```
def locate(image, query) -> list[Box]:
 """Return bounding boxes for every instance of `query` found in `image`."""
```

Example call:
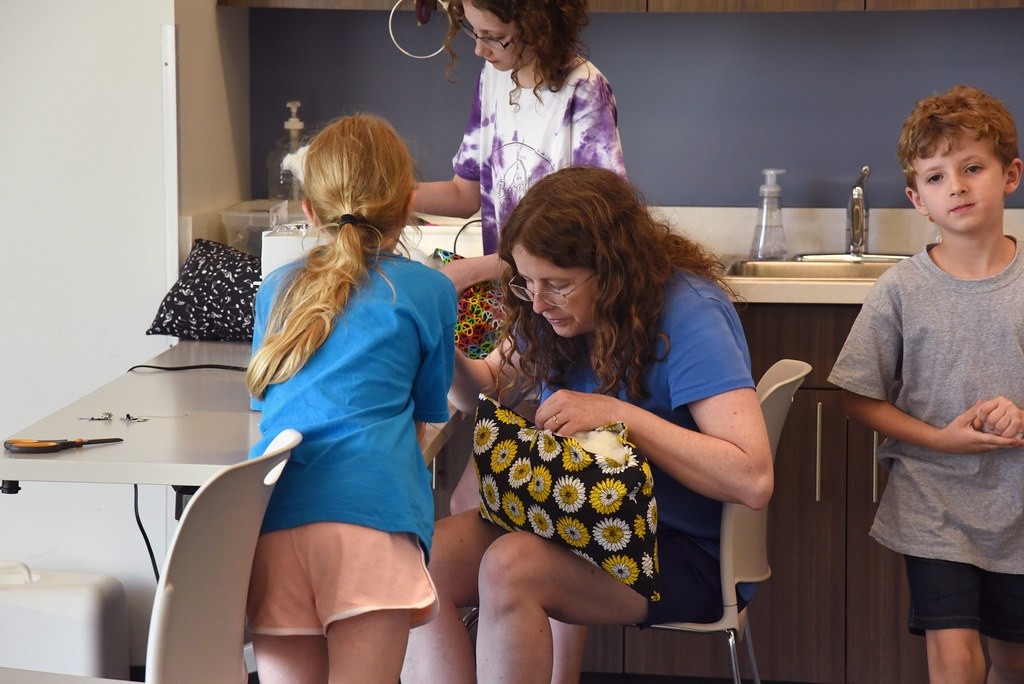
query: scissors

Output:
[4,437,123,454]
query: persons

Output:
[825,83,1024,684]
[246,113,459,683]
[398,167,774,684]
[404,0,630,684]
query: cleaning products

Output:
[265,100,320,233]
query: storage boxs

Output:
[220,198,303,255]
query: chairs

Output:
[0,428,301,684]
[633,358,812,684]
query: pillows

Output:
[144,237,261,341]
[473,393,662,602]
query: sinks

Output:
[722,258,900,281]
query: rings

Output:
[552,415,562,426]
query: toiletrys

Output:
[749,169,788,261]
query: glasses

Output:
[457,12,516,50]
[509,272,599,306]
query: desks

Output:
[0,341,465,494]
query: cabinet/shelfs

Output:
[579,303,929,684]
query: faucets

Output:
[844,165,870,257]
